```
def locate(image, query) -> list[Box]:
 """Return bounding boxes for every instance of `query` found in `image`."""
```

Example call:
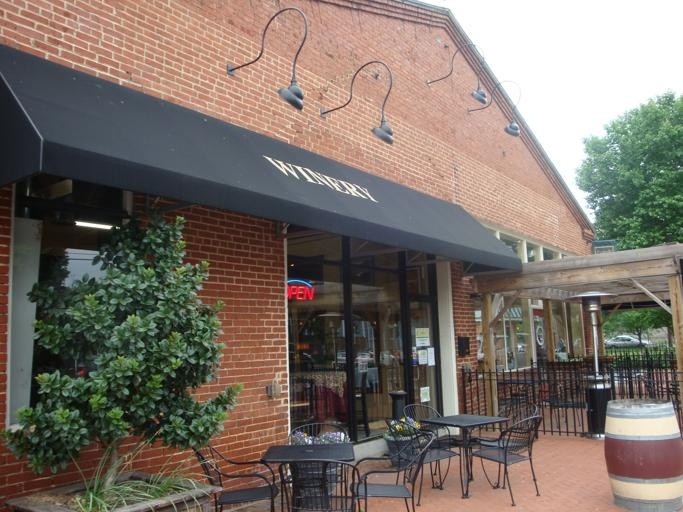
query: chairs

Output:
[350,433,435,511]
[384,403,542,507]
[192,421,368,511]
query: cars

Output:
[604,335,647,349]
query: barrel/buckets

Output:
[604,398,682,512]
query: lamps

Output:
[319,61,394,145]
[426,42,487,106]
[467,80,521,136]
[227,7,307,111]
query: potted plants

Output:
[0,196,222,512]
